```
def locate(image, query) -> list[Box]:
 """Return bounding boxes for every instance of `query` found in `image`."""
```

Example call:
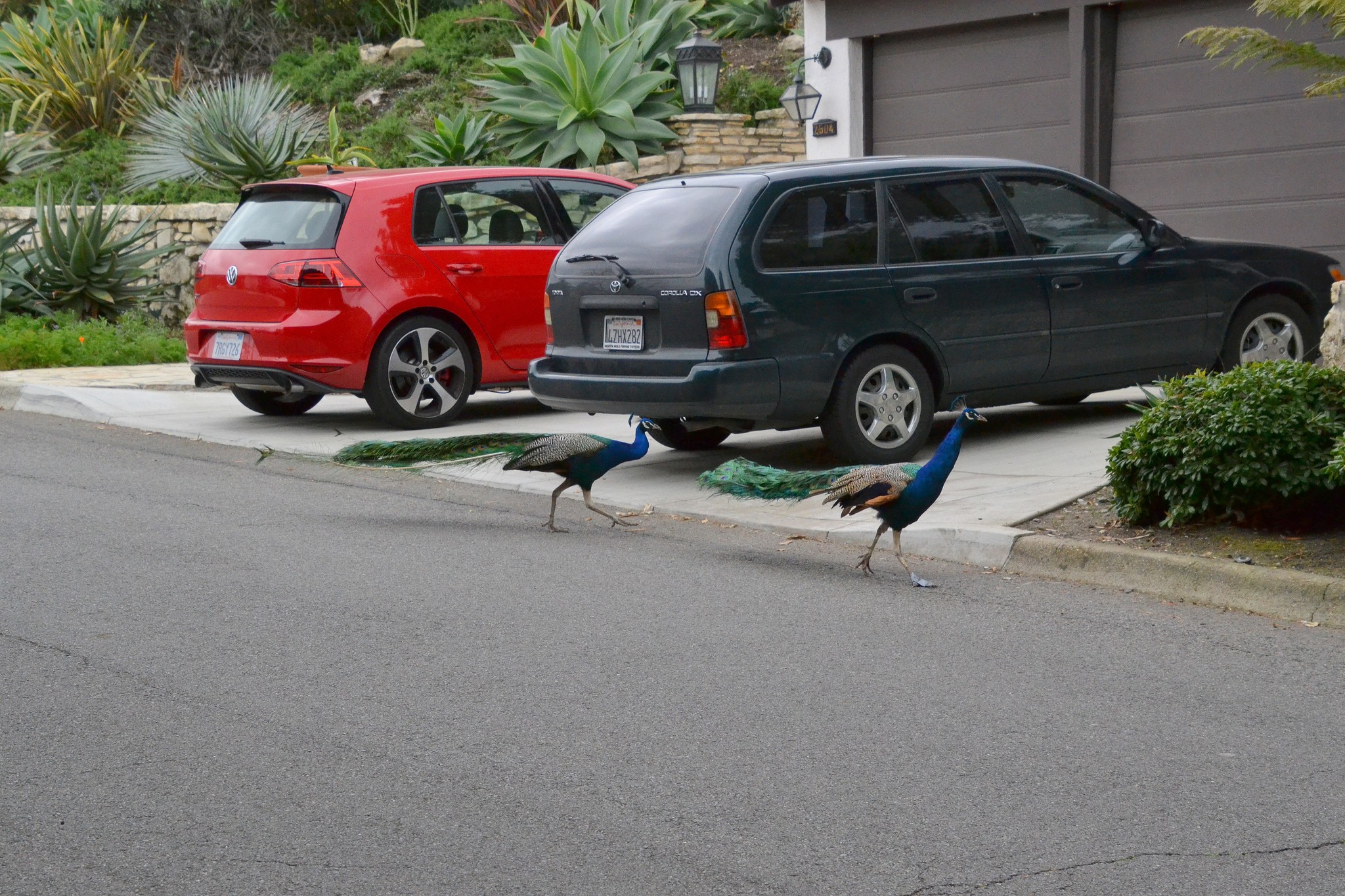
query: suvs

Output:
[526,152,1345,467]
[181,163,639,430]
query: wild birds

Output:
[695,395,988,588]
[254,409,661,533]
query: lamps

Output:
[777,47,833,124]
[670,27,726,115]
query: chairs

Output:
[482,206,526,246]
[301,208,332,238]
[431,201,472,242]
